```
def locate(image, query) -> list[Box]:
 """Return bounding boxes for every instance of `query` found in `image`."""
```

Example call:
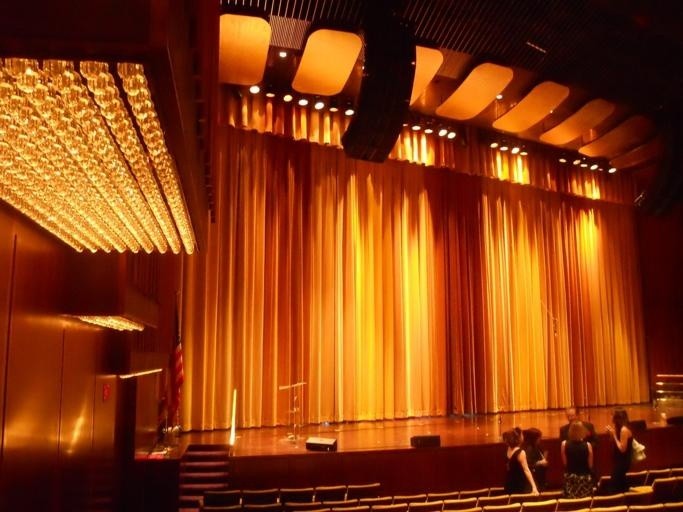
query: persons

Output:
[560,419,595,499]
[561,406,600,461]
[503,427,540,496]
[603,407,633,493]
[523,428,548,493]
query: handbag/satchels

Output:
[632,439,646,463]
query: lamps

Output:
[266,81,528,156]
[558,151,616,173]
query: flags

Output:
[174,308,184,412]
